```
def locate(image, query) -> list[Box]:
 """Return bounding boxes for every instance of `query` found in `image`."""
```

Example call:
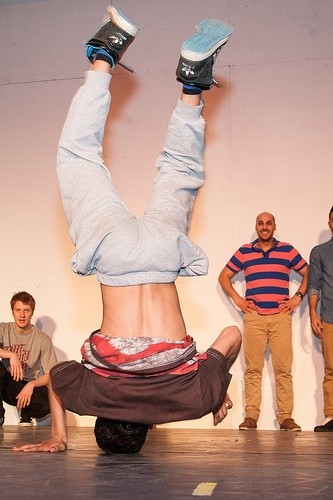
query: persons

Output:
[308,206,333,432]
[11,6,242,455]
[0,292,58,426]
[219,213,308,431]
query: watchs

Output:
[295,292,303,299]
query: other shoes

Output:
[17,408,34,427]
[280,418,303,431]
[174,18,234,90]
[238,418,258,430]
[0,418,5,427]
[314,420,333,432]
[85,4,140,70]
